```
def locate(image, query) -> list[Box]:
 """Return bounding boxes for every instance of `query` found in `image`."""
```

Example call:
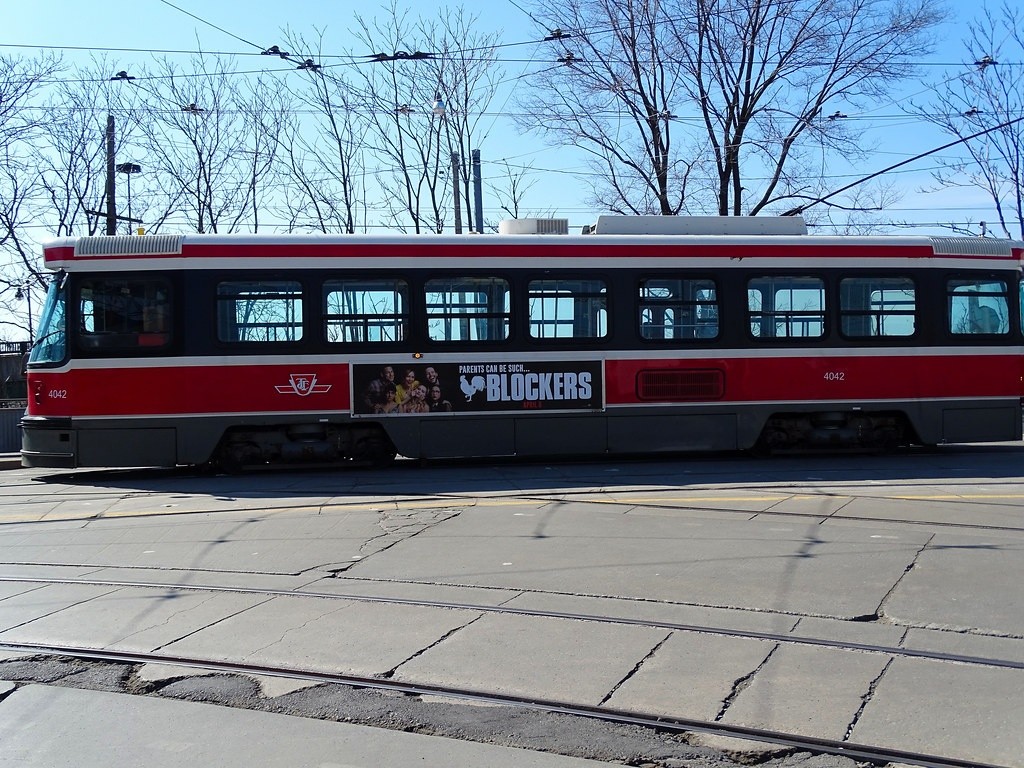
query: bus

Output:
[16,117,1024,472]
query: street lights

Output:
[116,162,142,235]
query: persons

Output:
[358,365,451,415]
